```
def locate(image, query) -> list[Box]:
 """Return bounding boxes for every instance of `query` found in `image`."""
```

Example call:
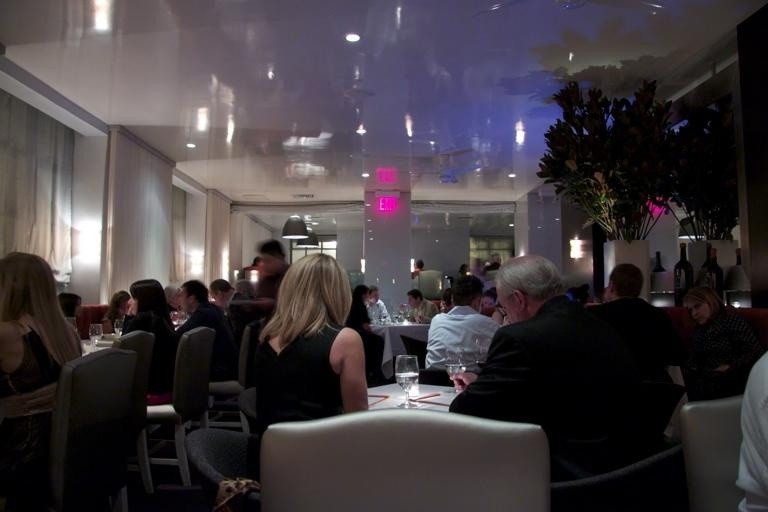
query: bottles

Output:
[652,250,666,272]
[699,241,724,301]
[673,243,693,306]
[736,247,741,265]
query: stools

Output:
[551,444,682,488]
[184,428,260,501]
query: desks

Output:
[80,334,118,356]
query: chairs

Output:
[258,410,552,512]
[679,396,742,512]
[112,330,155,495]
[147,327,216,487]
[209,318,263,432]
[47,347,138,512]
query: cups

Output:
[65,317,78,332]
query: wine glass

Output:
[443,346,468,377]
[114,319,125,336]
[393,353,421,409]
[88,324,104,351]
[373,303,424,325]
[471,335,493,366]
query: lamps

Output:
[281,216,319,246]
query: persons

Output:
[411,260,424,279]
[121,279,177,406]
[58,293,82,323]
[346,284,391,335]
[238,253,368,435]
[425,275,510,374]
[165,287,181,312]
[210,238,290,343]
[736,352,767,512]
[450,254,638,512]
[406,289,439,322]
[0,252,82,498]
[583,264,688,379]
[682,286,767,403]
[101,291,131,334]
[180,281,239,395]
